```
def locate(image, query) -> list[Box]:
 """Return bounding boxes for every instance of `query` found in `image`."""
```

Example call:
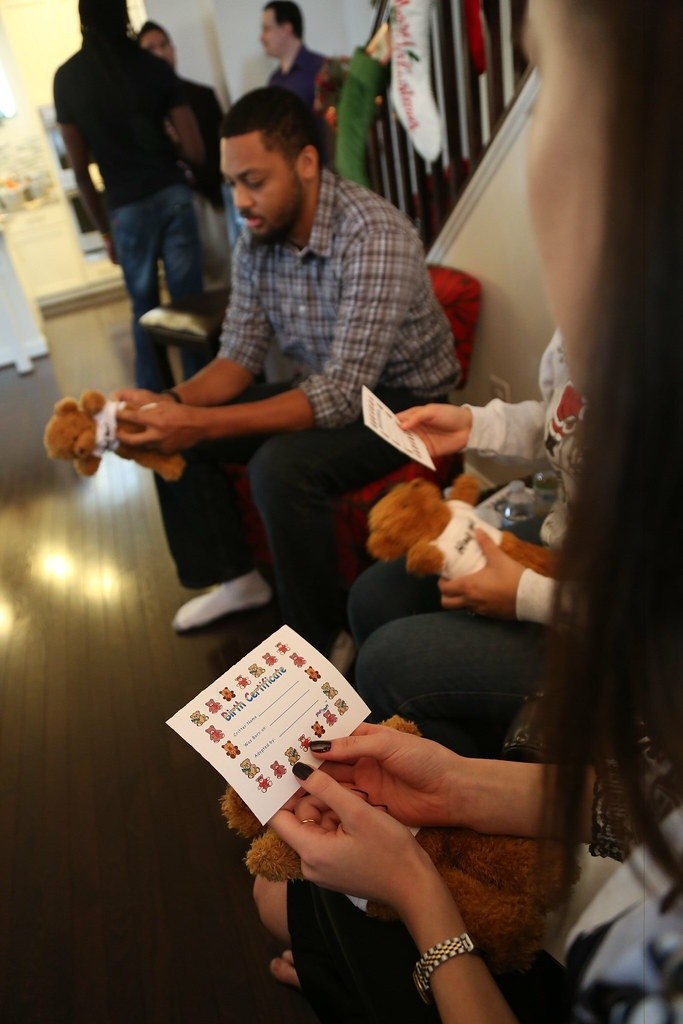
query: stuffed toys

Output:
[217,710,575,952]
[365,477,564,633]
[45,389,193,482]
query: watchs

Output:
[414,931,478,1005]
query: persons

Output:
[54,0,225,394]
[140,21,224,290]
[260,0,345,128]
[250,2,683,1022]
[131,86,467,635]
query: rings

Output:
[299,817,317,825]
